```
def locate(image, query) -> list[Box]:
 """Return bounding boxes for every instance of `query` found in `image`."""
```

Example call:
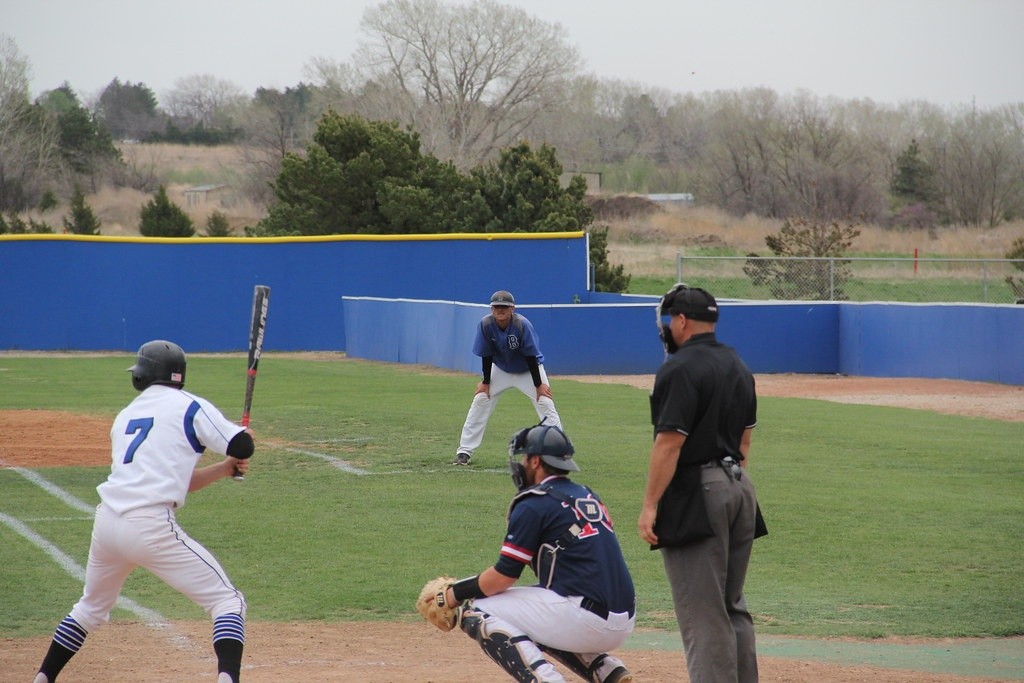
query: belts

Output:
[710,459,737,467]
[581,598,634,621]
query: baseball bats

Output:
[232,284,271,482]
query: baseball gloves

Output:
[415,575,461,632]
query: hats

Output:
[663,289,718,322]
[489,290,515,306]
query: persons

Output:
[453,289,562,467]
[638,282,769,683]
[32,340,255,683]
[415,416,637,683]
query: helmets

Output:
[523,426,581,472]
[126,340,186,390]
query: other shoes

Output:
[603,666,633,683]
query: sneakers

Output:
[453,453,470,465]
